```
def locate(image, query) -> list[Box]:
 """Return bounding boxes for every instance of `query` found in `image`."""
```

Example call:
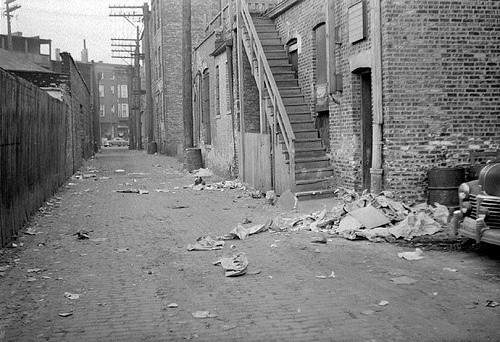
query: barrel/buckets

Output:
[187,148,202,173]
[427,167,465,215]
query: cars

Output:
[107,138,129,147]
[450,162,499,246]
